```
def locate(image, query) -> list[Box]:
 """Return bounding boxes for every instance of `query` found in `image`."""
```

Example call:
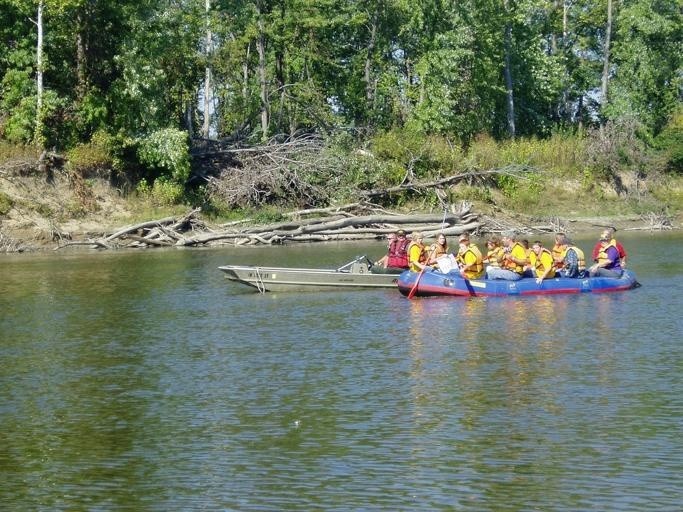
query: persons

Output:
[374,229,627,284]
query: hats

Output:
[397,230,406,236]
[459,234,469,242]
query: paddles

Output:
[408,248,434,298]
[460,272,486,289]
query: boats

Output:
[395,266,641,298]
[217,262,405,294]
